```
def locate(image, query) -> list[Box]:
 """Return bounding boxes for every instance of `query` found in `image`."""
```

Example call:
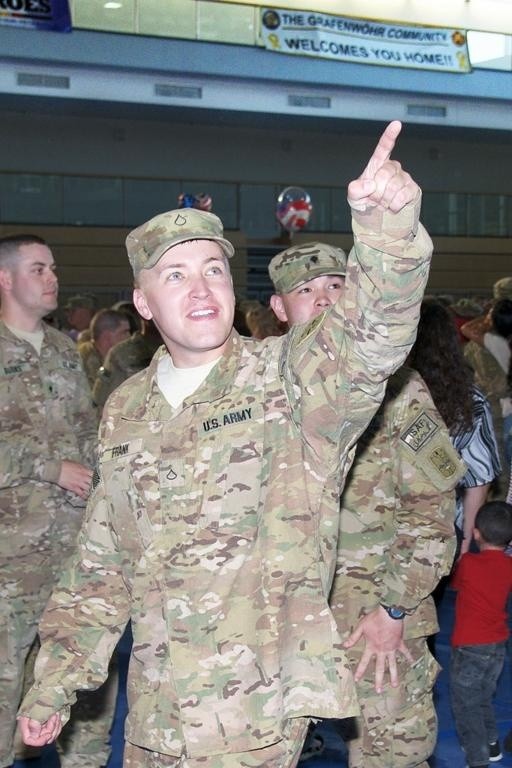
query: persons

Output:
[405,275,511,767]
[15,120,459,768]
[44,295,284,407]
[1,234,119,767]
[268,244,459,767]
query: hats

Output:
[124,207,235,271]
[493,278,512,300]
[64,298,96,310]
[268,242,346,296]
[449,299,483,318]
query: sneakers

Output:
[299,734,324,761]
[487,741,503,763]
